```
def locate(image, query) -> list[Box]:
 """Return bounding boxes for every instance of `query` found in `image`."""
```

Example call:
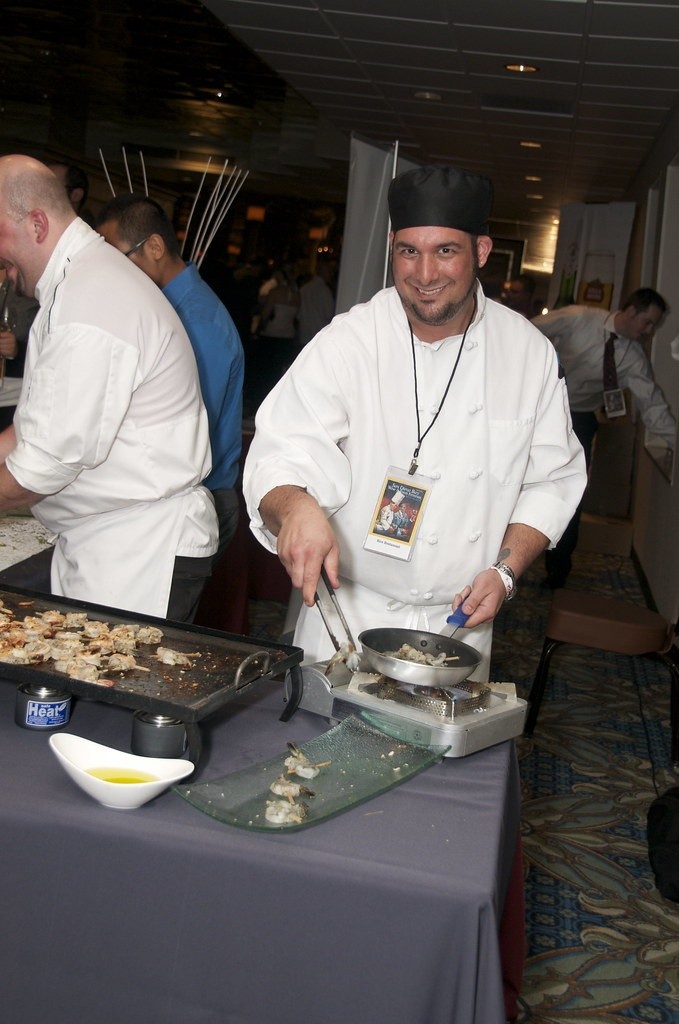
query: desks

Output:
[0,664,521,1023]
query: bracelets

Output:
[493,561,517,601]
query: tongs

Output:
[314,565,358,673]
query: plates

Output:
[170,712,452,834]
[48,733,195,809]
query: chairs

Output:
[520,588,679,763]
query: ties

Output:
[603,332,620,422]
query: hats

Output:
[387,165,492,237]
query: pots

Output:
[357,603,485,688]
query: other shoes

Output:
[541,566,570,591]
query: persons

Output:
[510,275,536,315]
[255,251,338,369]
[241,168,586,687]
[530,288,677,595]
[374,490,417,538]
[94,195,245,621]
[0,162,88,431]
[0,154,219,626]
